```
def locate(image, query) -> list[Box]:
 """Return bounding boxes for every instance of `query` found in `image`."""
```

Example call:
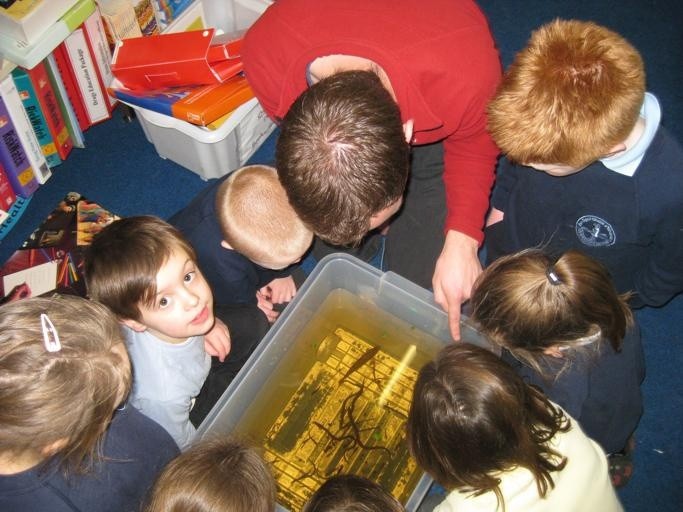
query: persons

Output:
[166,162,316,325]
[407,338,624,511]
[304,472,409,512]
[239,0,505,342]
[138,431,282,511]
[0,291,183,512]
[80,211,232,450]
[479,17,683,311]
[468,244,647,456]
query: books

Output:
[1,0,255,212]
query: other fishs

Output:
[312,359,393,477]
[338,345,381,386]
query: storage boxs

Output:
[183,251,500,509]
[125,1,285,186]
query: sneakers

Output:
[607,436,634,489]
